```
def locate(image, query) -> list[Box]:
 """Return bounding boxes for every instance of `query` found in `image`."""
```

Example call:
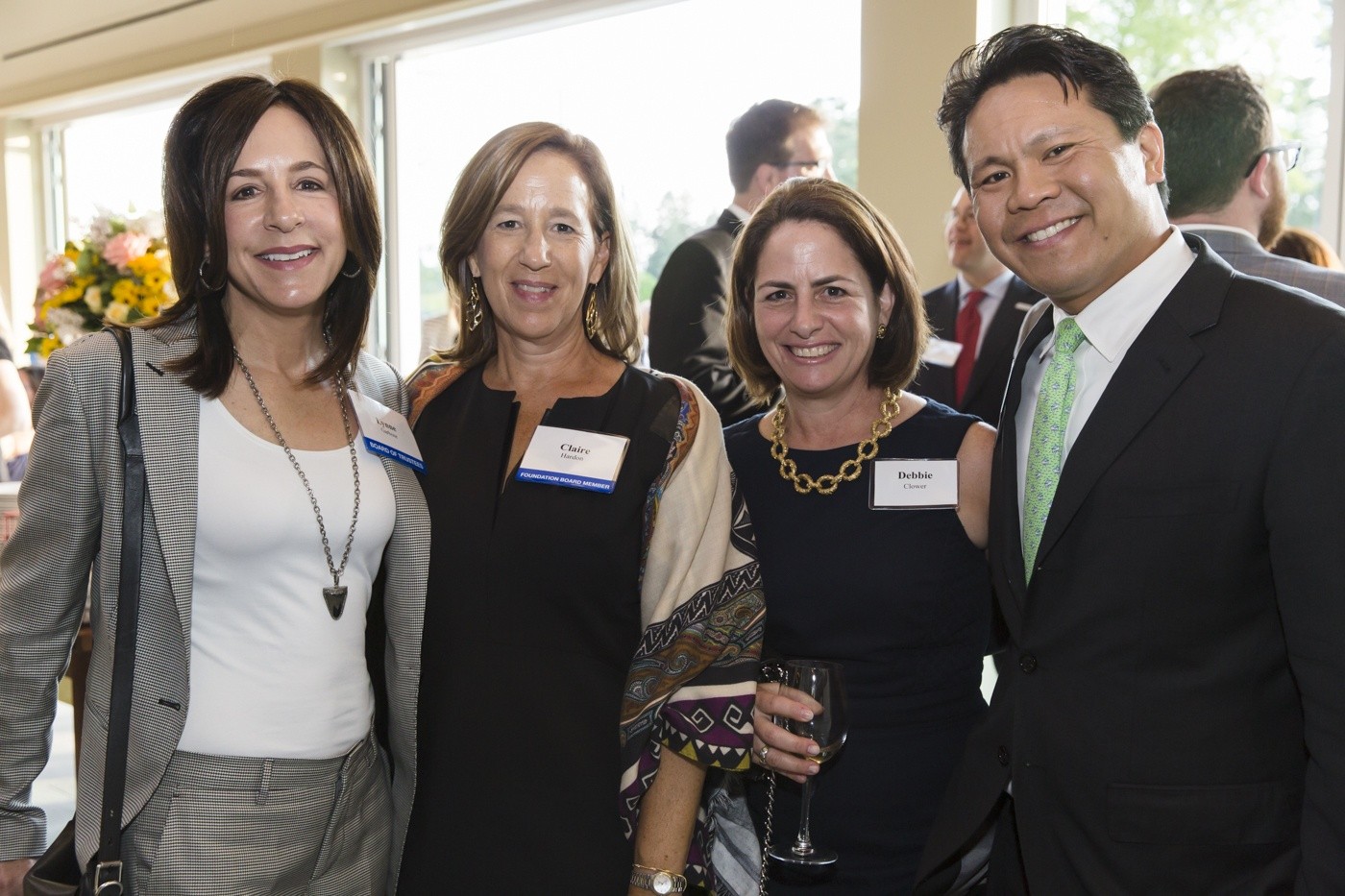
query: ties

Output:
[953,291,988,409]
[1021,316,1087,587]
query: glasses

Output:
[770,159,833,176]
[1244,143,1300,179]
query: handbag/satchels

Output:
[21,813,127,896]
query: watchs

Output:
[630,862,688,896]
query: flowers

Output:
[27,211,180,368]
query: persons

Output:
[0,77,433,896]
[649,100,840,416]
[900,183,1043,424]
[695,177,1019,895]
[404,119,767,896]
[933,21,1345,896]
[1145,64,1345,307]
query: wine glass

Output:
[764,660,848,866]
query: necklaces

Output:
[767,380,903,501]
[230,316,360,624]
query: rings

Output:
[758,744,770,765]
